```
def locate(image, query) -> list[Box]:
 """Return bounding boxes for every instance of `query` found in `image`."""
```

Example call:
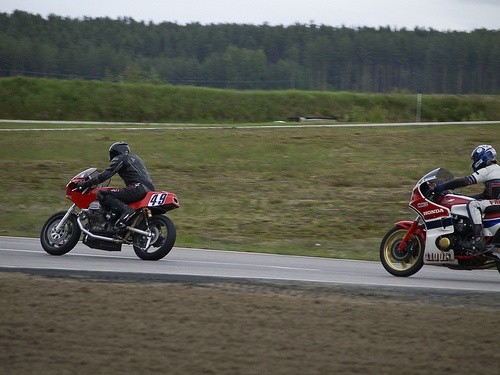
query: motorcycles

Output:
[379,167,500,277]
[40,168,180,260]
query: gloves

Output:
[426,183,443,195]
[77,180,93,192]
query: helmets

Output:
[470,144,497,172]
[108,142,131,160]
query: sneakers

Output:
[461,232,484,247]
[115,207,135,227]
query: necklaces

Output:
[426,143,499,250]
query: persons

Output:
[71,140,154,229]
